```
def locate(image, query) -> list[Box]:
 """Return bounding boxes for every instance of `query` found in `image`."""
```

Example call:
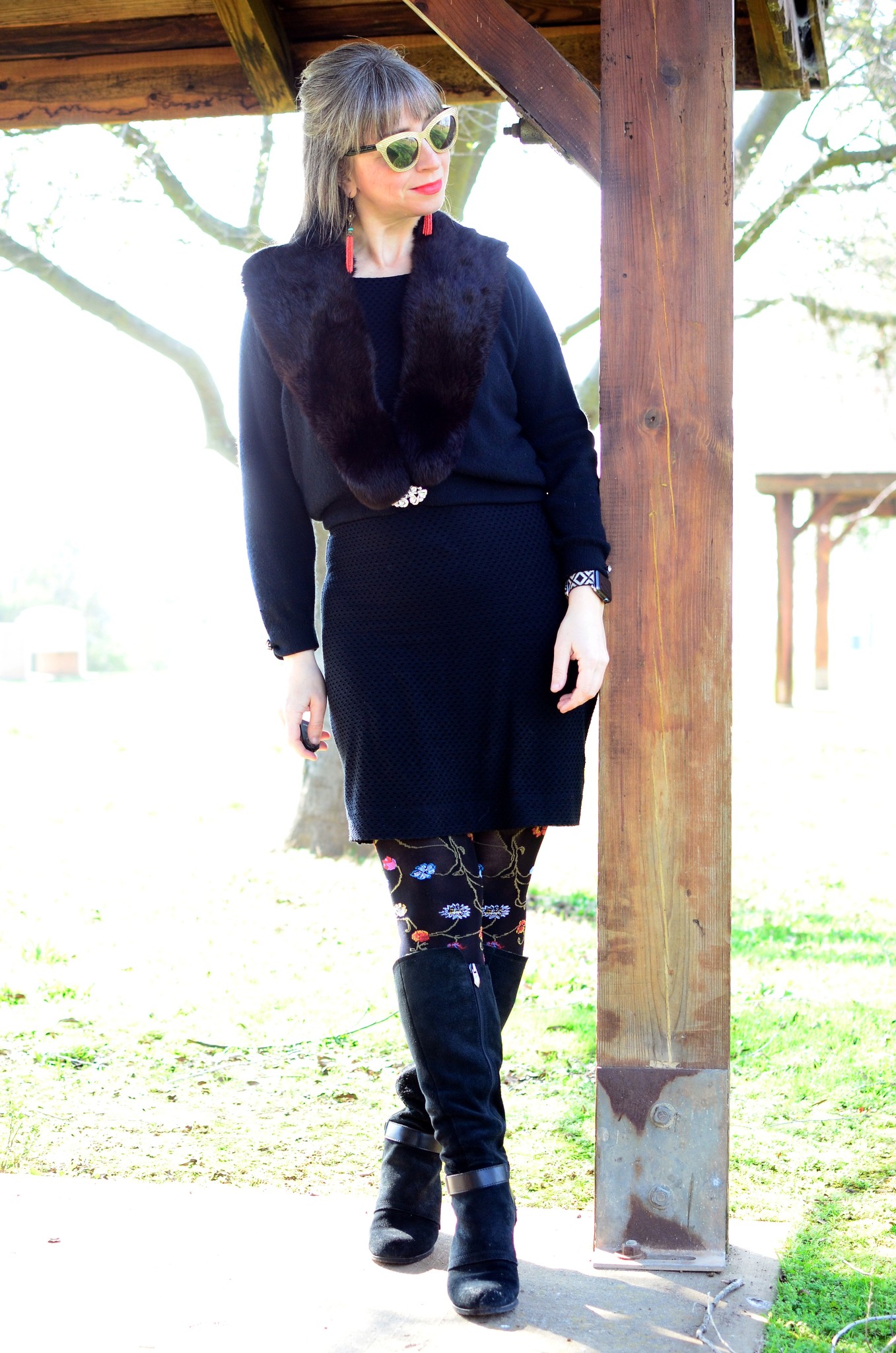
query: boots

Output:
[372,944,526,1265]
[393,944,521,1316]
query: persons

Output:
[238,43,612,1321]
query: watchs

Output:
[565,570,612,604]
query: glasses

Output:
[341,104,460,173]
